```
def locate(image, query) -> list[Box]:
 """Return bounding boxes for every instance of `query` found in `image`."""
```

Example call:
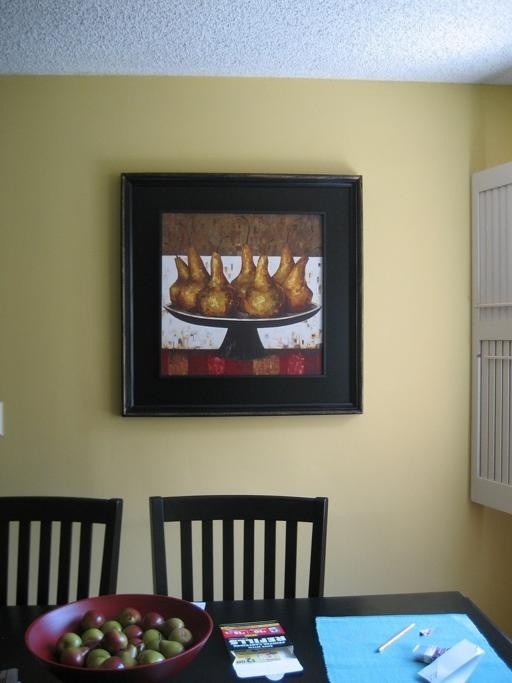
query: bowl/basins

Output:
[23,594,215,681]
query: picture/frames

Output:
[120,171,362,417]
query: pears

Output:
[170,243,313,317]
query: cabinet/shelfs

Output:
[470,160,512,515]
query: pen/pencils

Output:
[377,623,415,653]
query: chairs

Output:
[148,495,329,601]
[0,496,123,606]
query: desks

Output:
[0,591,512,683]
